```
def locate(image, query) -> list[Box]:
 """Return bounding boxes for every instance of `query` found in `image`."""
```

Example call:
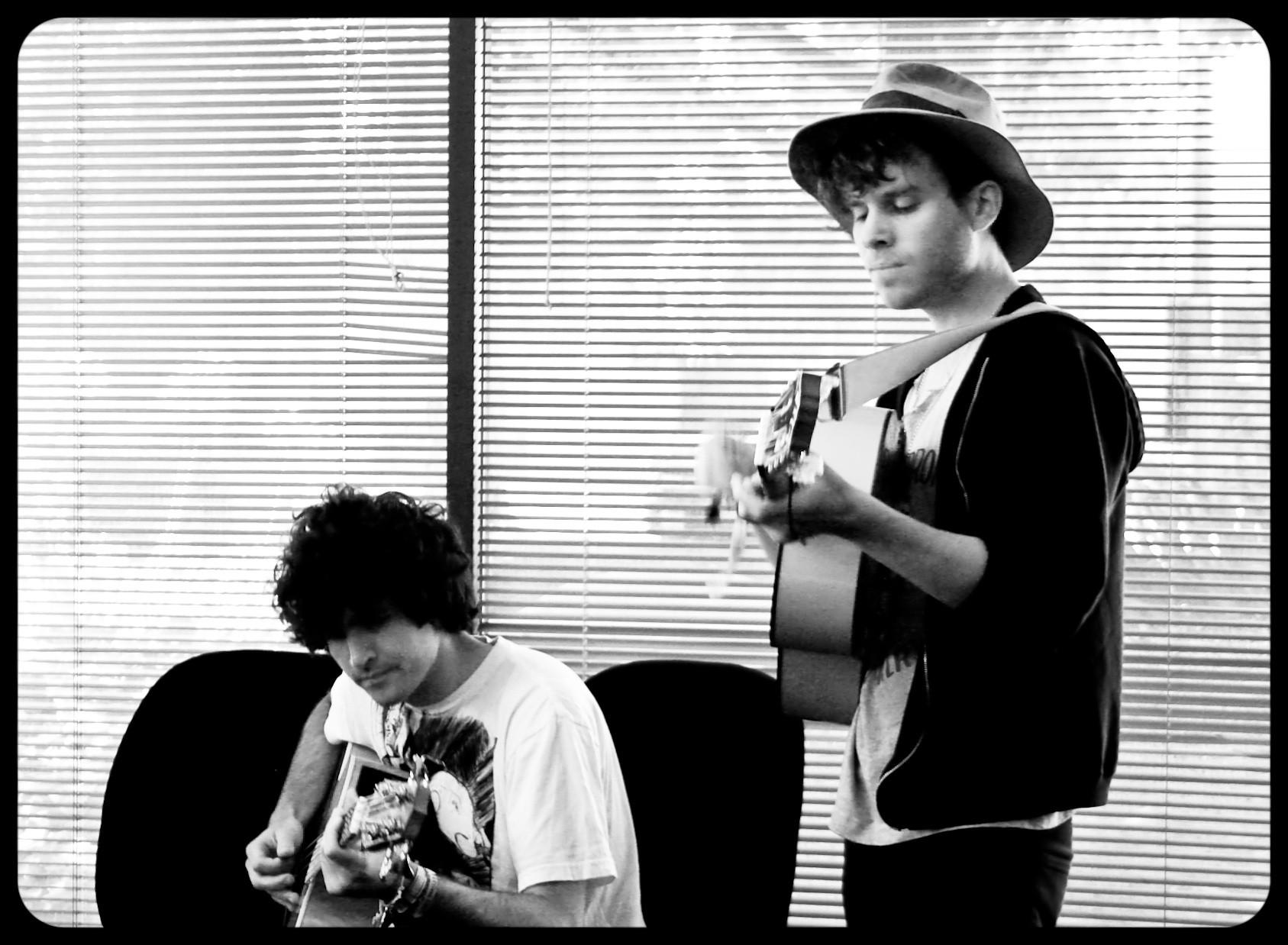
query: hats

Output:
[788,65,1053,270]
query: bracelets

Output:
[405,866,438,911]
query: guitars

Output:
[282,739,433,934]
[752,370,900,726]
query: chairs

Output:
[95,649,344,933]
[586,660,805,929]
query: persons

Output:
[246,483,646,927]
[695,63,1147,927]
